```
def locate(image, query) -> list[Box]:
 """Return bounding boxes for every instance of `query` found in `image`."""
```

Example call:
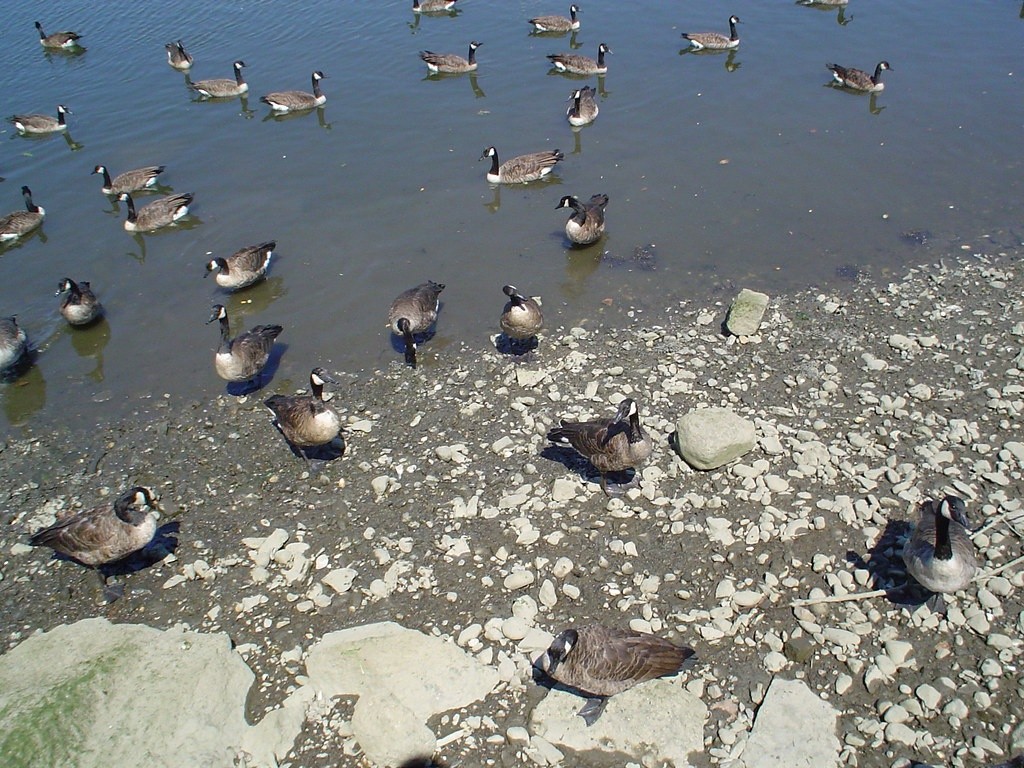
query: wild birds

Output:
[682,14,745,50]
[794,0,848,5]
[537,622,697,727]
[1,0,655,606]
[825,60,895,91]
[902,492,978,615]
